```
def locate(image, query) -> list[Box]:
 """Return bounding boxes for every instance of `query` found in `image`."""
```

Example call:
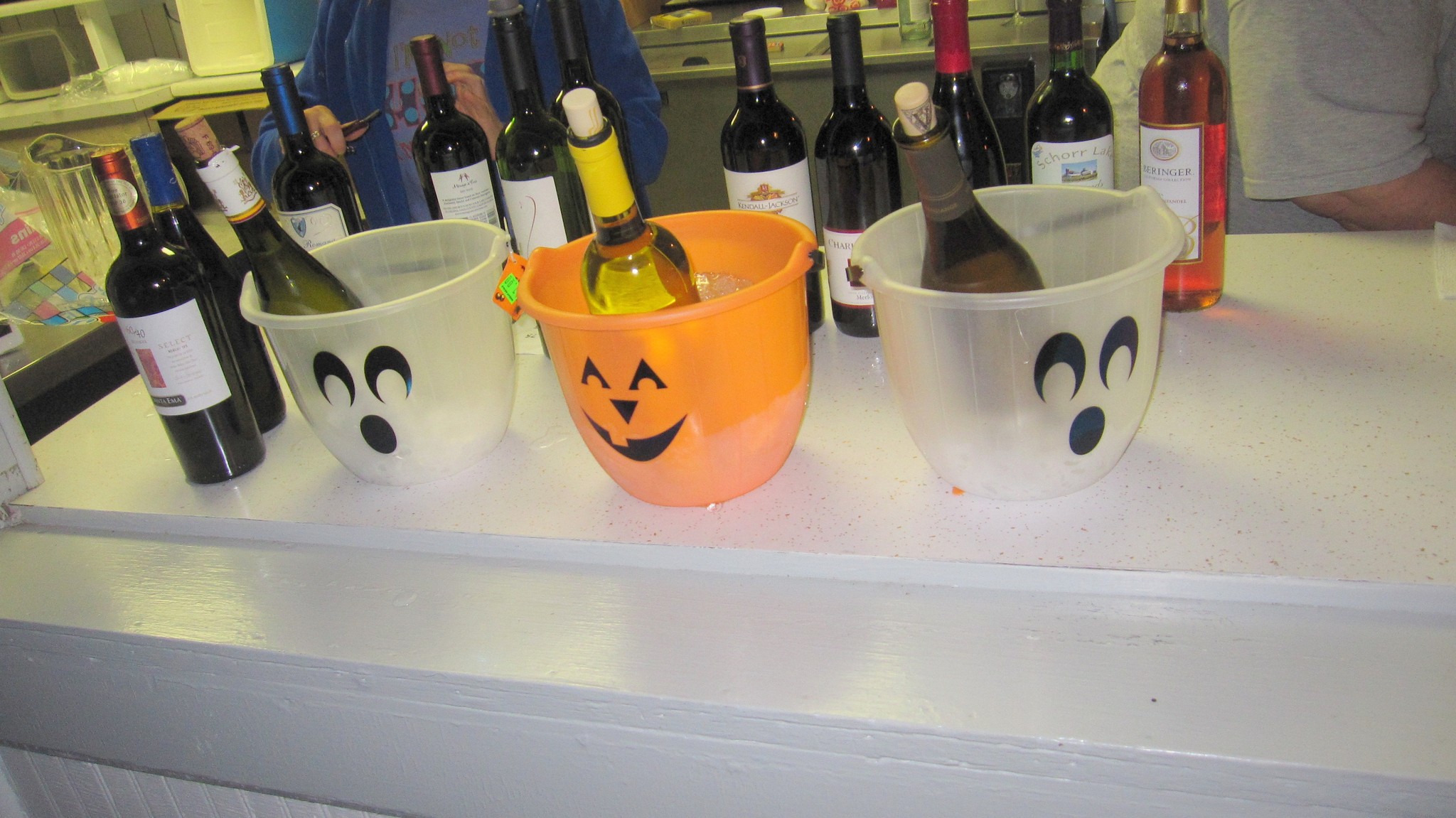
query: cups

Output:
[19,133,189,308]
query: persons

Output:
[1091,0,1456,235]
[251,0,668,230]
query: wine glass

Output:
[1002,0,1033,28]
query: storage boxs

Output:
[649,8,712,31]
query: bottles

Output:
[930,0,1009,191]
[174,112,363,315]
[720,17,825,334]
[90,147,265,484]
[548,0,641,237]
[260,62,366,253]
[813,11,903,337]
[897,0,932,40]
[409,35,513,270]
[487,0,594,360]
[1138,0,1228,313]
[562,88,701,314]
[1025,0,1115,194]
[891,81,1045,292]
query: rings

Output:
[311,130,322,140]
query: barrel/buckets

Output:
[517,210,825,507]
[845,183,1185,502]
[239,220,515,485]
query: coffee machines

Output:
[129,134,285,435]
